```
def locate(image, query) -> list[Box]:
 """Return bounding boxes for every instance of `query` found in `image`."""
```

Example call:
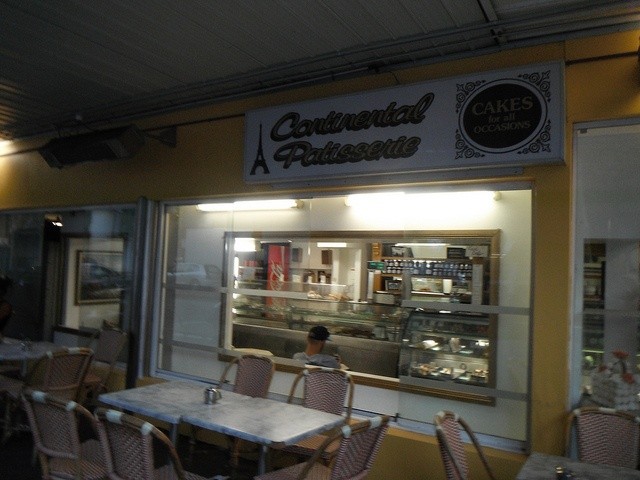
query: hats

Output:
[308,326,333,342]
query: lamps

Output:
[344,190,503,205]
[195,199,305,211]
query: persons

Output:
[292,326,341,369]
[0,277,13,333]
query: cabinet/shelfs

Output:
[395,311,497,389]
[380,256,489,317]
[289,241,342,311]
[235,252,266,317]
[233,292,415,378]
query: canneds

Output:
[381,259,471,279]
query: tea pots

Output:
[204,386,222,405]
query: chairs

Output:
[0,346,94,444]
[254,415,390,479]
[434,410,494,480]
[94,405,206,480]
[20,388,109,480]
[564,405,640,471]
[279,367,355,464]
[187,355,275,467]
[77,329,127,406]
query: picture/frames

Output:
[73,249,124,307]
